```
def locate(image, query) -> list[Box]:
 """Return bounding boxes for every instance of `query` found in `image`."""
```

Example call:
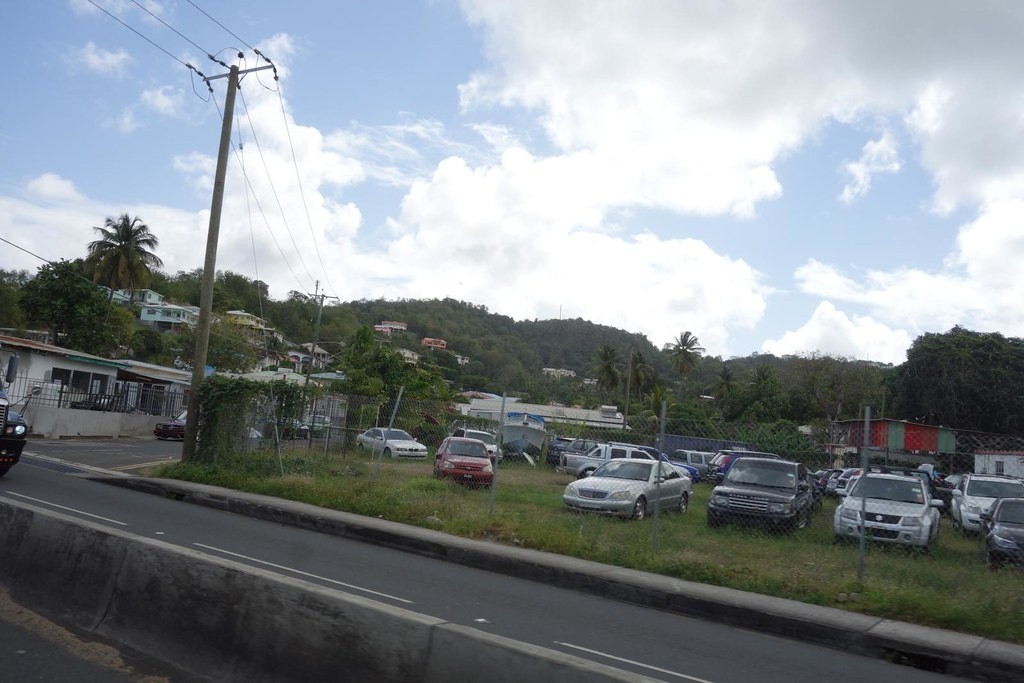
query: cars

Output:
[639,446,701,482]
[355,427,429,462]
[433,428,504,488]
[562,458,694,522]
[265,415,334,441]
[70,395,150,415]
[950,472,1024,566]
[814,466,946,547]
[153,408,206,443]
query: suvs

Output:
[545,437,603,466]
[705,448,826,534]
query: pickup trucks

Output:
[561,444,693,483]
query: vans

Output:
[672,449,715,479]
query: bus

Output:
[0,341,42,476]
